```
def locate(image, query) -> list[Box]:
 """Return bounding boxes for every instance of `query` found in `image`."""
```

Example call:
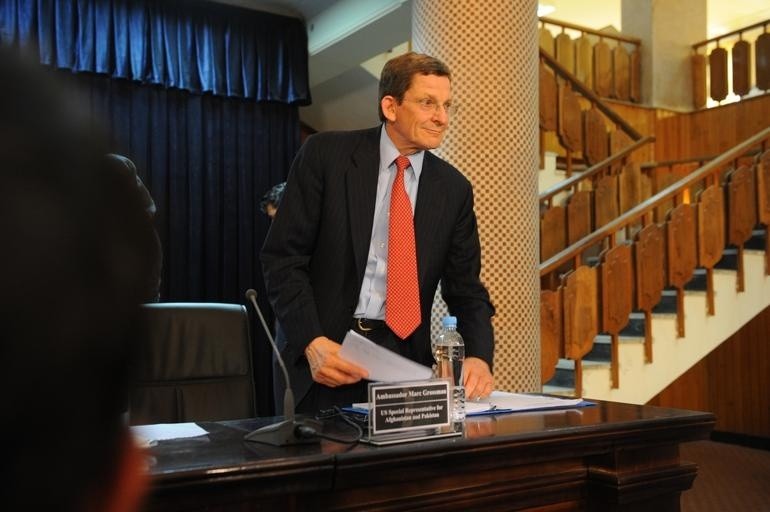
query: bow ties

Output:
[384,156,422,341]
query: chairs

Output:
[128,303,257,424]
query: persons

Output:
[257,49,499,422]
[255,181,291,223]
[0,35,161,512]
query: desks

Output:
[130,394,716,512]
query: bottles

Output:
[432,315,465,424]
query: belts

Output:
[349,318,391,332]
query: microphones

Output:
[245,289,324,448]
